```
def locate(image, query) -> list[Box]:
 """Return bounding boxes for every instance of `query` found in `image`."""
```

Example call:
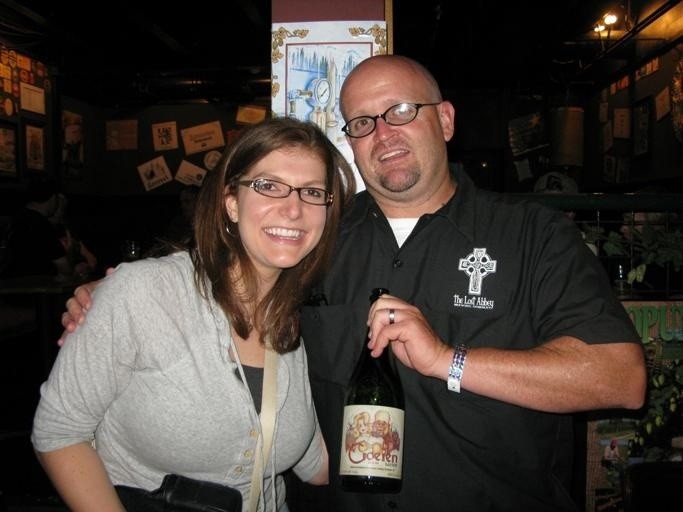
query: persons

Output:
[609,185,682,301]
[28,116,357,511]
[0,170,97,286]
[532,170,625,260]
[141,185,202,260]
[50,54,648,512]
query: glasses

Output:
[342,103,439,137]
[240,179,334,205]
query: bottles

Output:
[336,288,405,495]
[128,241,139,260]
[611,260,632,303]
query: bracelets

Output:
[446,341,470,395]
